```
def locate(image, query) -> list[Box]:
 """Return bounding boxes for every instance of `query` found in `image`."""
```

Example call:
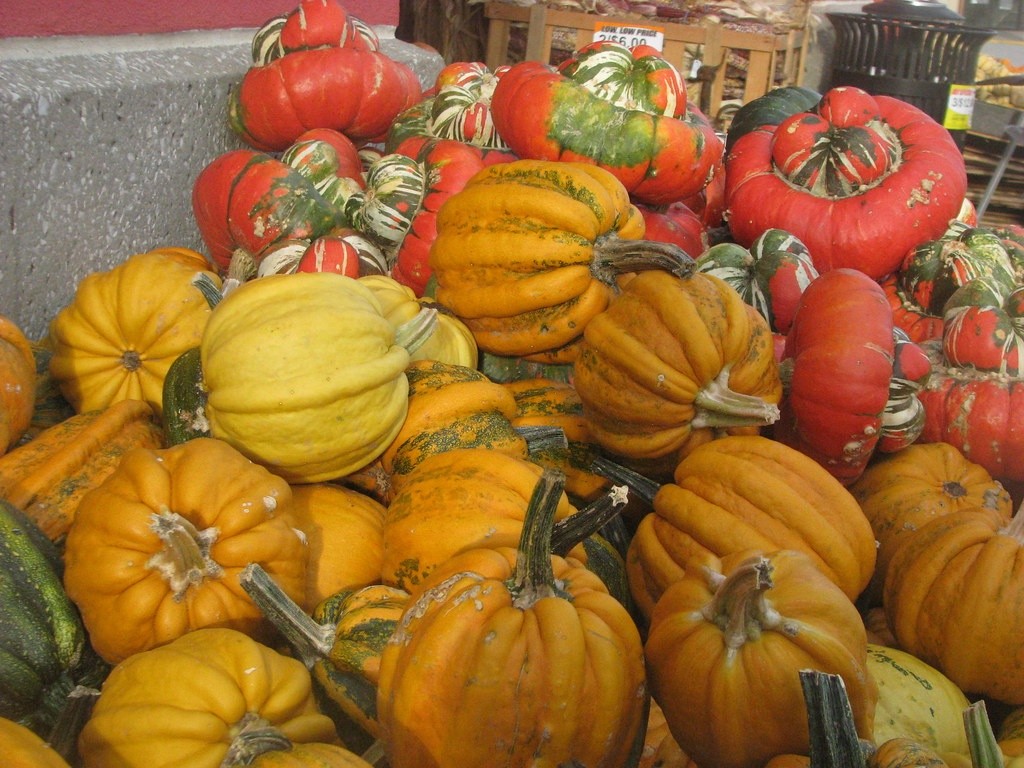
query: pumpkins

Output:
[0,0,1024,768]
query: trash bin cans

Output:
[824,12,997,157]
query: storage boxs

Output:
[482,3,809,132]
